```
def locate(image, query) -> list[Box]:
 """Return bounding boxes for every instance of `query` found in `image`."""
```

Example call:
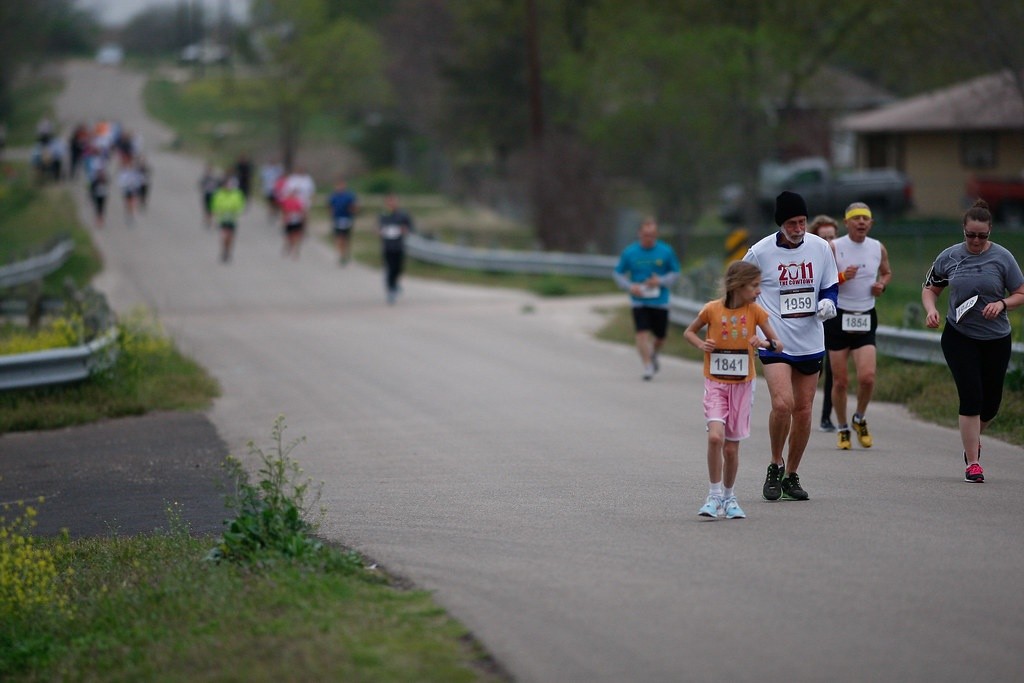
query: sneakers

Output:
[837,428,851,450]
[763,460,785,500]
[851,414,873,448]
[965,464,984,482]
[783,472,808,501]
[963,435,981,465]
[698,496,722,518]
[723,497,746,518]
[820,418,835,432]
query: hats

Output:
[774,191,808,228]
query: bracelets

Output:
[880,282,886,293]
[997,299,1006,312]
[839,272,847,285]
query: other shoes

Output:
[650,357,658,371]
[641,362,653,378]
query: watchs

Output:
[765,338,776,352]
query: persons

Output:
[34,116,151,226]
[806,214,838,432]
[829,202,891,449]
[198,152,317,265]
[329,179,358,262]
[612,215,680,378]
[684,261,784,518]
[740,190,838,500]
[921,198,1024,482]
[379,193,411,302]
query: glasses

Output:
[965,228,991,239]
